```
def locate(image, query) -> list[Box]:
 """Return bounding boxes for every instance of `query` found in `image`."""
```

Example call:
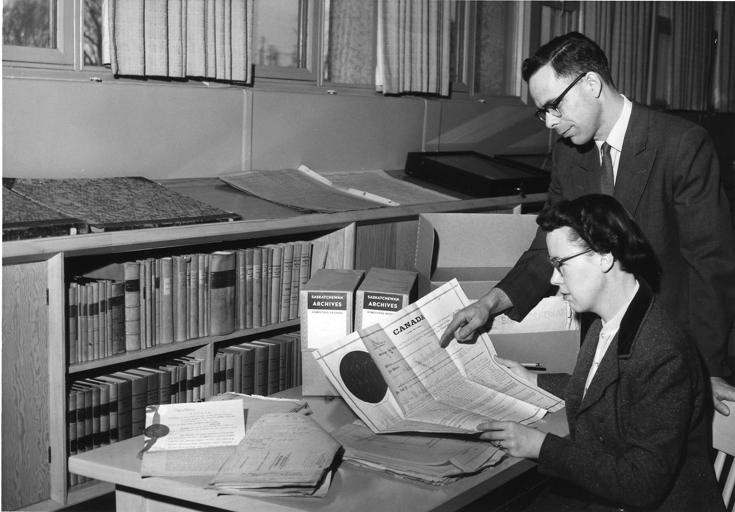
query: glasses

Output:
[551,249,593,269]
[535,73,585,122]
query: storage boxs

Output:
[415,210,582,375]
[297,267,365,397]
[353,267,417,375]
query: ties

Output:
[597,142,614,195]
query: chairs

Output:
[709,399,735,511]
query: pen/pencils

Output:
[520,361,545,368]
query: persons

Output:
[475,194,728,511]
[439,32,735,418]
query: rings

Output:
[498,440,505,450]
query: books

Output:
[67,242,313,366]
[67,330,301,487]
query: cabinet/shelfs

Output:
[1,168,548,512]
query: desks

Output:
[67,373,572,512]
